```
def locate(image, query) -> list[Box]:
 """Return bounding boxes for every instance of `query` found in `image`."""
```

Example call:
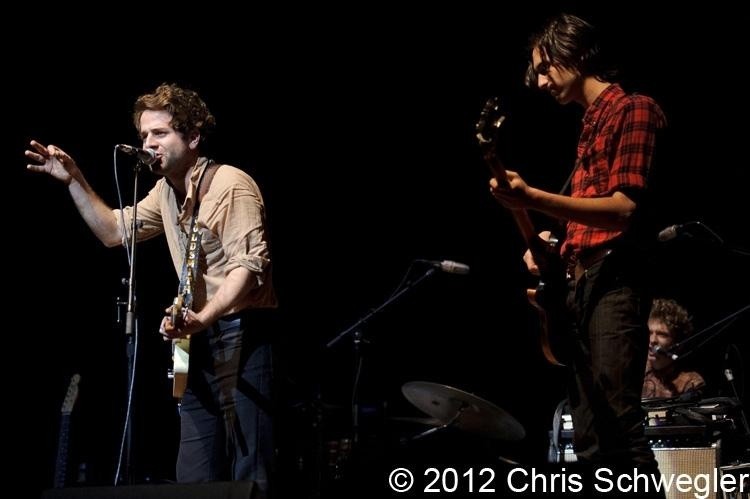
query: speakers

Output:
[43,480,265,499]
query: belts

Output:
[574,248,611,280]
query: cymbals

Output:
[402,381,526,442]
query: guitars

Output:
[478,96,578,366]
[52,373,79,490]
[170,297,191,399]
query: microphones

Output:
[724,369,734,381]
[653,345,679,361]
[658,224,684,241]
[440,260,470,275]
[119,144,156,165]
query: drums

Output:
[305,432,363,497]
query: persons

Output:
[25,83,279,498]
[488,17,671,498]
[642,298,707,400]
